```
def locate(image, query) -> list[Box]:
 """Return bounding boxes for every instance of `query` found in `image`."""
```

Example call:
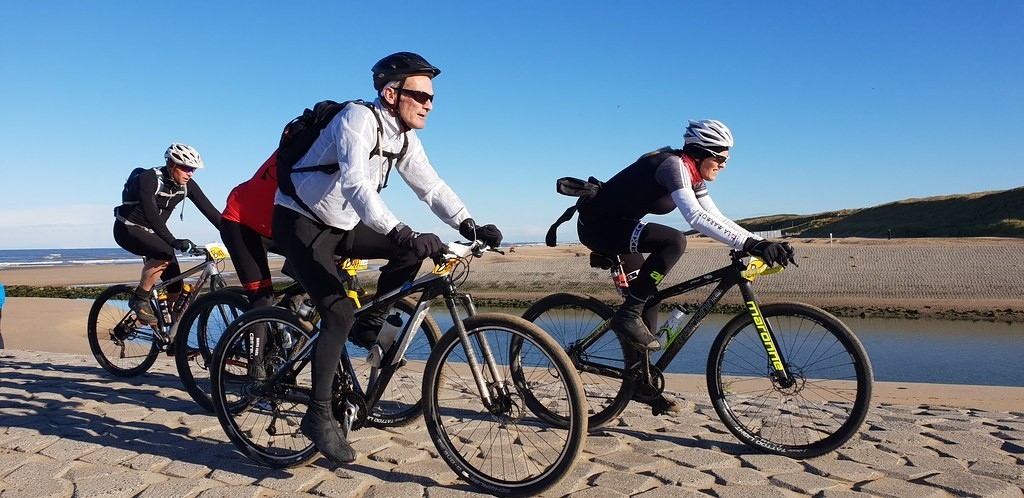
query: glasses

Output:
[394,88,434,104]
[694,145,730,163]
[177,165,196,173]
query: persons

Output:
[577,119,796,411]
[220,148,279,389]
[272,51,503,464]
[113,143,221,358]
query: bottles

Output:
[366,312,404,368]
[655,303,690,348]
[157,290,174,327]
[276,319,292,348]
[173,283,193,313]
[297,299,312,317]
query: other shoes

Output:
[300,397,357,463]
[166,341,199,356]
[128,293,158,325]
[247,364,270,380]
[348,318,408,366]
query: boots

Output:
[626,359,681,411]
[611,292,661,352]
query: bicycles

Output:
[87,236,259,379]
[510,240,874,458]
[208,222,588,498]
[174,254,448,429]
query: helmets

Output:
[371,52,441,90]
[164,143,205,169]
[683,119,734,148]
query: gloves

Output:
[459,218,503,248]
[386,222,448,258]
[173,238,202,256]
[743,237,800,269]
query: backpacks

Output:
[276,98,409,197]
[122,166,187,205]
